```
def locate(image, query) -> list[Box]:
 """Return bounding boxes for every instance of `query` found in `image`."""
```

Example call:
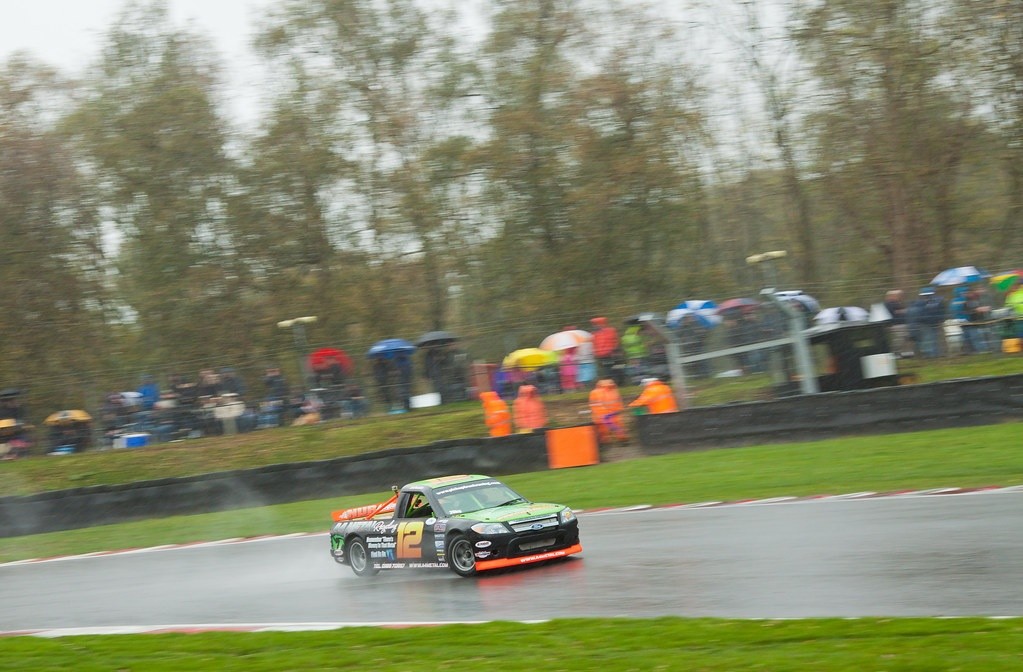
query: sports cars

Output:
[328,474,582,579]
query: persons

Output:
[512,384,546,432]
[911,287,944,358]
[311,359,352,413]
[103,367,289,447]
[947,284,1023,353]
[884,290,910,358]
[373,350,413,404]
[590,378,631,446]
[478,391,512,436]
[494,314,710,396]
[630,378,678,413]
[424,341,473,402]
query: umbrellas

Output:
[501,347,546,369]
[0,417,23,428]
[308,348,353,373]
[42,409,92,426]
[416,331,459,346]
[715,299,760,316]
[929,265,989,286]
[667,300,722,330]
[367,338,418,357]
[540,329,591,352]
